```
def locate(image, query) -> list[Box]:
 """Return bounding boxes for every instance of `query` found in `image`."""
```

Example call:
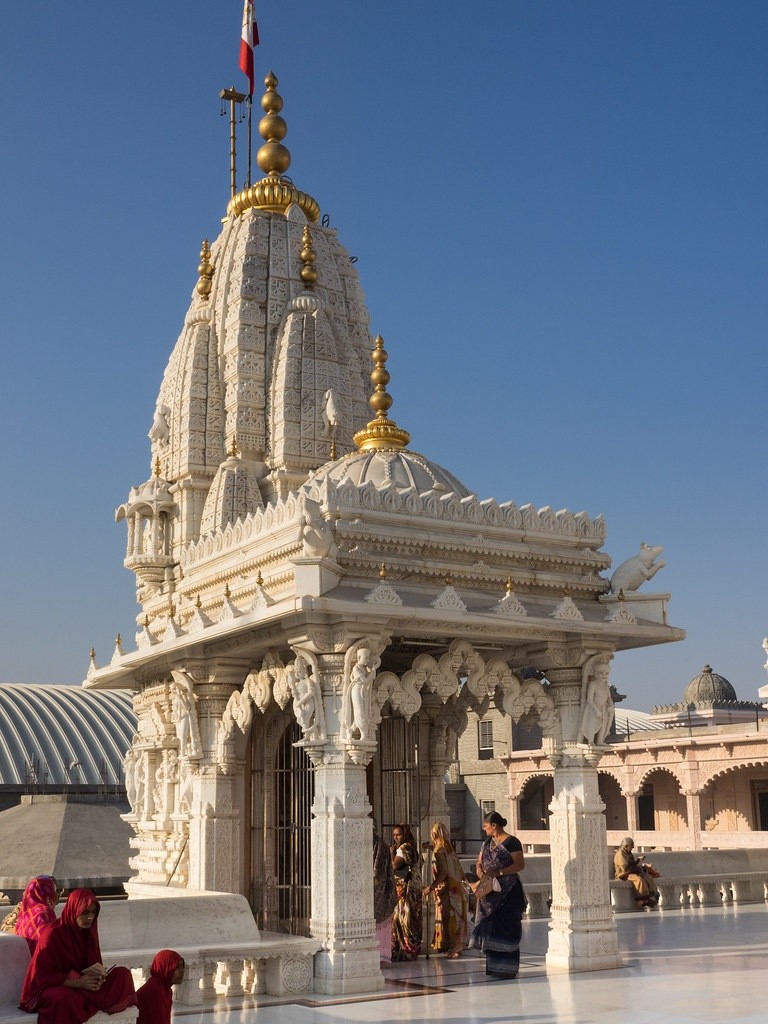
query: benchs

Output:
[0,883,320,1006]
[0,936,139,1024]
[523,846,768,919]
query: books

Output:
[81,962,116,977]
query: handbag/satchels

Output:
[461,876,492,900]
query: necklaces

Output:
[490,836,498,851]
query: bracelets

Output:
[477,868,481,875]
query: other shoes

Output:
[446,945,463,959]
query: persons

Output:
[475,811,526,979]
[134,950,185,1024]
[388,822,422,961]
[613,836,661,909]
[372,819,393,965]
[21,888,136,1024]
[423,823,468,960]
[4,873,59,952]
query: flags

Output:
[241,0,260,95]
